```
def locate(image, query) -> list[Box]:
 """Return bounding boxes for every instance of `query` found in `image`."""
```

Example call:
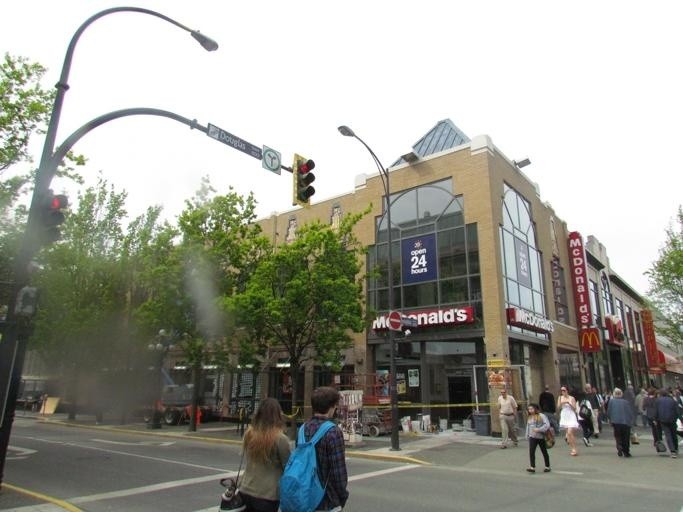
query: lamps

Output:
[511,155,532,171]
[395,149,421,165]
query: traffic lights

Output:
[292,151,316,210]
[36,188,69,245]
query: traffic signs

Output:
[400,317,417,328]
[205,121,262,160]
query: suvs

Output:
[139,382,210,426]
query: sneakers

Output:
[501,432,678,472]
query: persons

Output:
[524,382,683,474]
[237,397,291,512]
[294,385,350,512]
[496,389,519,449]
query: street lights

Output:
[0,2,222,486]
[147,329,175,429]
[336,124,402,452]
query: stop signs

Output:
[387,309,401,331]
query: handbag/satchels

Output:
[580,404,591,418]
[219,487,247,512]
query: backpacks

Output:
[279,421,336,511]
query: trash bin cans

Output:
[473,414,490,436]
